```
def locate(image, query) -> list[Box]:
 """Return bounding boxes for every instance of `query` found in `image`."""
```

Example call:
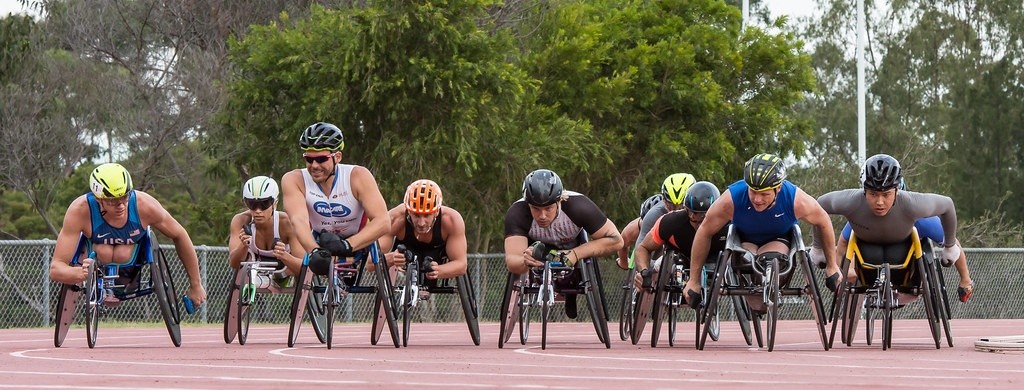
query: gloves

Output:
[318,229,352,257]
[308,247,332,276]
[809,247,826,266]
[942,245,961,267]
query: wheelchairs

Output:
[827,226,957,351]
[53,224,184,349]
[498,228,612,350]
[619,223,829,352]
[223,239,330,348]
[372,245,481,348]
[287,239,401,349]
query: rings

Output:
[277,250,279,253]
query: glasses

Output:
[244,196,274,211]
[303,151,340,164]
[103,193,131,206]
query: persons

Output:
[810,154,976,310]
[49,163,206,310]
[614,173,767,316]
[228,176,308,278]
[503,168,624,273]
[681,152,843,309]
[365,179,468,279]
[280,122,392,277]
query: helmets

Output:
[684,180,721,212]
[522,169,561,206]
[403,178,442,215]
[662,173,696,205]
[859,153,901,196]
[299,122,344,152]
[744,152,787,191]
[640,194,663,220]
[89,162,133,199]
[241,176,279,208]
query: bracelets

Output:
[572,249,579,262]
